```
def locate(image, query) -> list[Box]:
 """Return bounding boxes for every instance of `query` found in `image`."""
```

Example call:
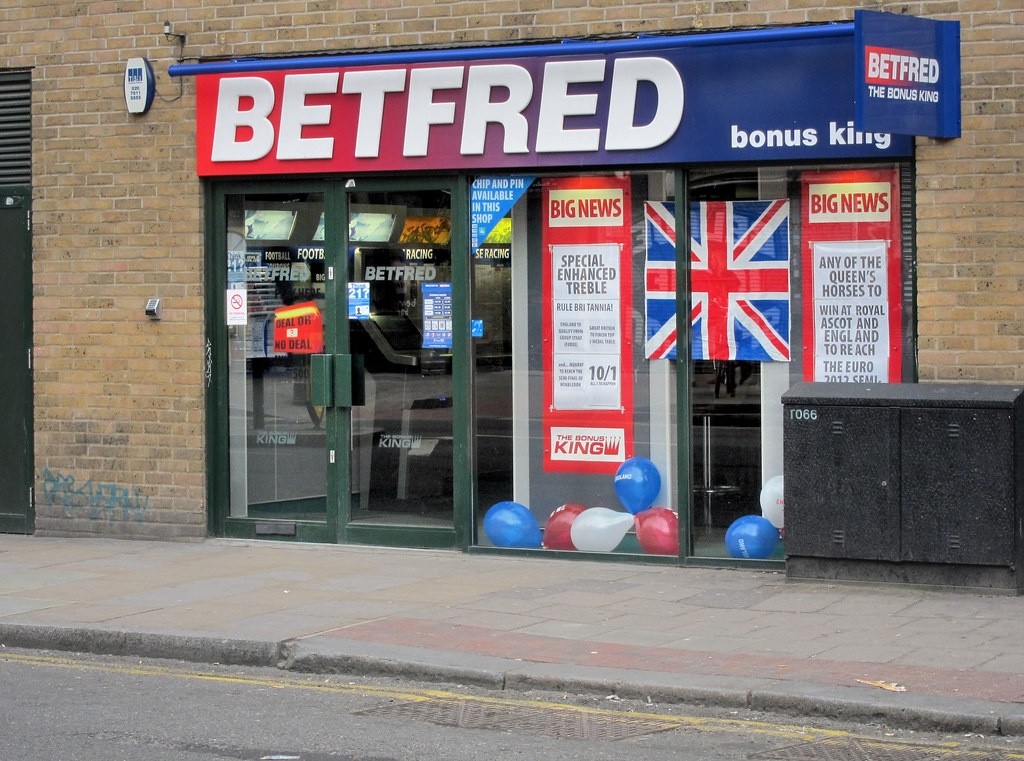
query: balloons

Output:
[635,507,679,555]
[543,503,588,548]
[760,475,783,529]
[570,507,633,552]
[483,501,542,548]
[725,515,778,559]
[614,458,660,514]
